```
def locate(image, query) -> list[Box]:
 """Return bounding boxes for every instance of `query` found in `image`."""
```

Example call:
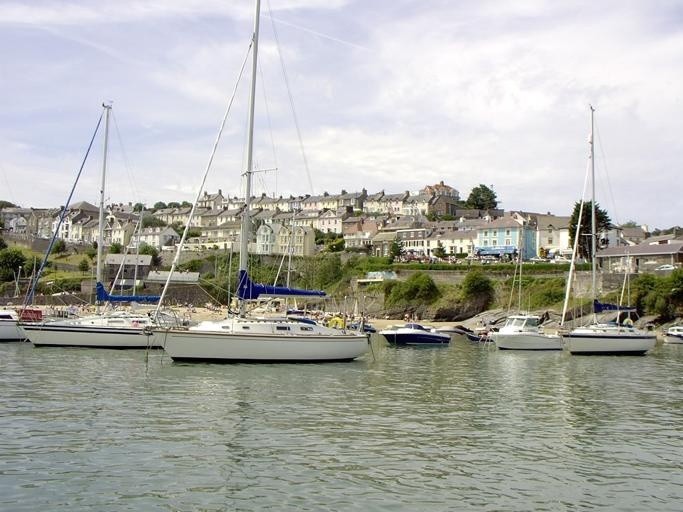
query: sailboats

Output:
[13,98,195,351]
[143,0,376,365]
[556,102,658,357]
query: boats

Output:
[378,313,452,348]
[1,264,378,344]
[463,228,683,352]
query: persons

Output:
[177,300,197,326]
[538,245,563,259]
[298,303,365,333]
[404,313,418,323]
[45,302,96,314]
[265,302,285,312]
[537,310,550,325]
[207,303,222,316]
[165,236,175,253]
[248,303,255,309]
[402,251,438,264]
[449,257,462,264]
[476,317,487,343]
[480,255,518,265]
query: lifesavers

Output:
[624,318,633,326]
